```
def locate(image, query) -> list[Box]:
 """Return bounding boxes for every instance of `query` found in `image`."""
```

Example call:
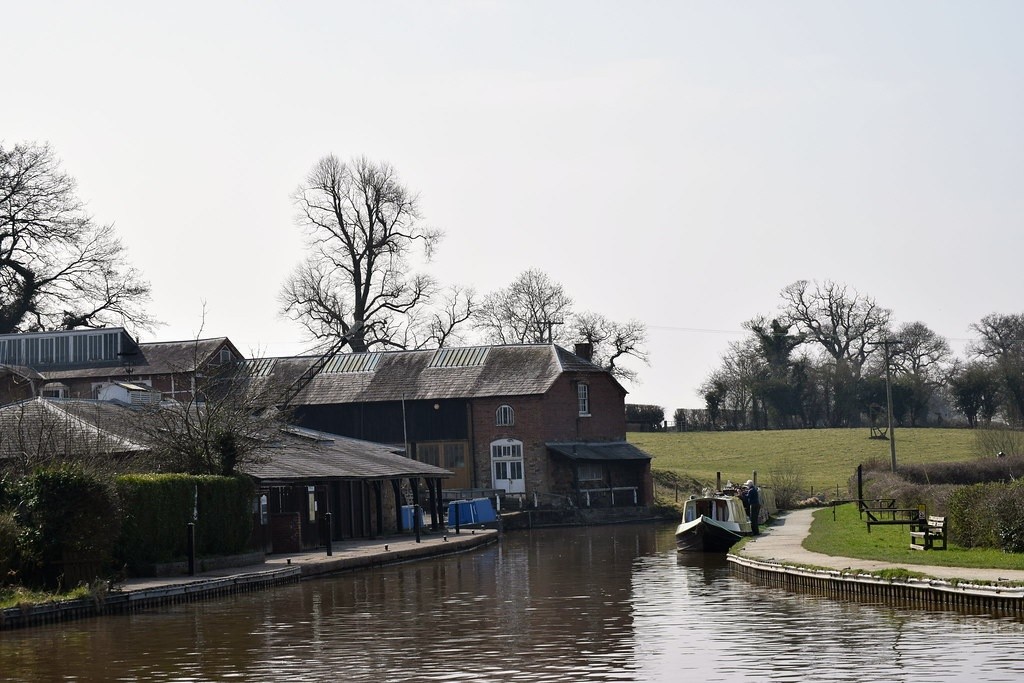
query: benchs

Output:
[865,508,919,534]
[857,498,896,520]
[910,515,947,551]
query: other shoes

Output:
[750,533,760,536]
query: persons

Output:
[747,480,760,536]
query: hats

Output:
[744,479,753,485]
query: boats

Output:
[675,479,753,553]
